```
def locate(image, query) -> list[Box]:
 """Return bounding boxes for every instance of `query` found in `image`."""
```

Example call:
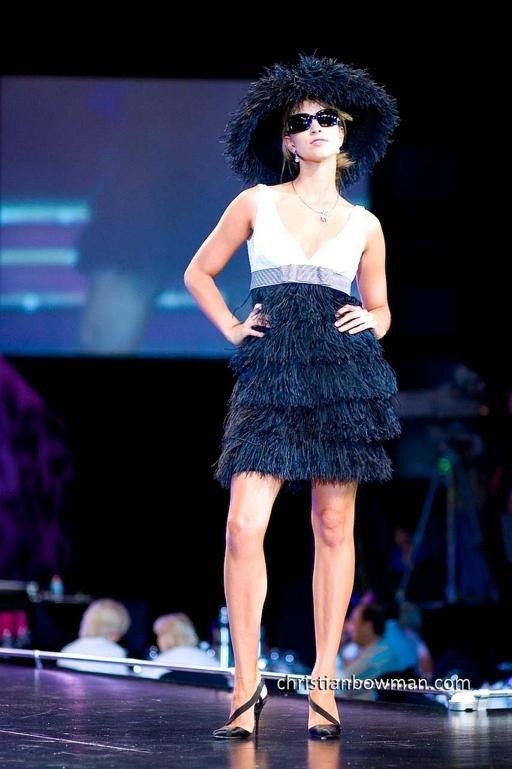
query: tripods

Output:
[398,459,497,608]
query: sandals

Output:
[303,675,344,738]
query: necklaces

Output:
[291,181,340,225]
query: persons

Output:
[360,589,435,684]
[337,598,420,698]
[180,51,410,743]
[54,596,132,673]
[132,610,222,682]
[385,523,415,573]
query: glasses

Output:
[280,107,346,138]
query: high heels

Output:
[213,678,273,741]
